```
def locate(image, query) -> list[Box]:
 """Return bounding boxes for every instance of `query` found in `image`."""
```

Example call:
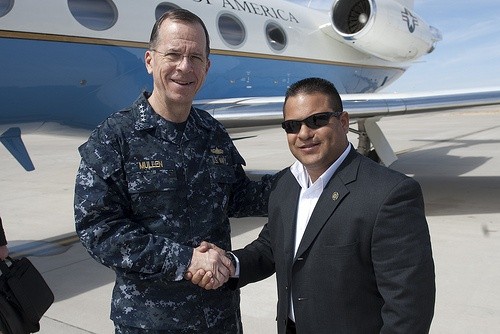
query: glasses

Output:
[153,48,206,67]
[282,112,342,134]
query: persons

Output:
[0,217,9,261]
[74,8,294,334]
[185,78,437,334]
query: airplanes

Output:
[0,0,500,260]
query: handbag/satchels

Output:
[0,256,54,334]
